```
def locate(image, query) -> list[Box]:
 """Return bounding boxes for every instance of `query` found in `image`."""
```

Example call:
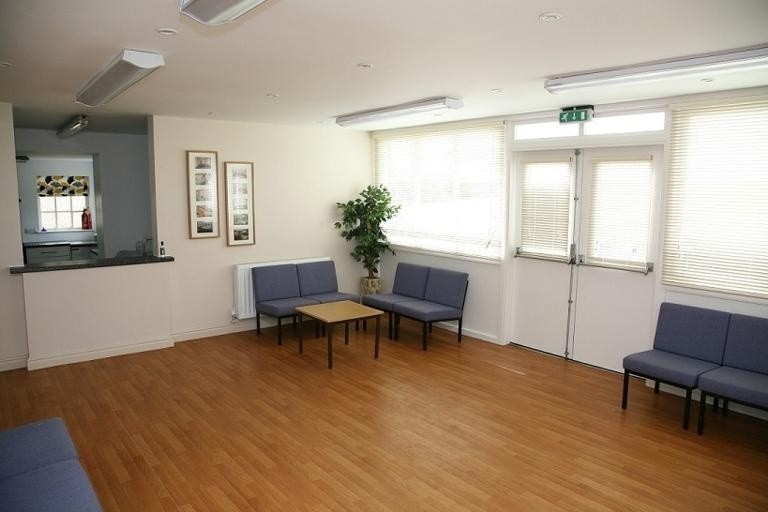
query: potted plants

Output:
[333,184,400,310]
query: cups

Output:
[135,240,145,254]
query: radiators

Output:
[228,254,333,324]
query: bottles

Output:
[82,208,90,230]
[160,241,165,257]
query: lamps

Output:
[336,96,466,136]
[544,52,767,105]
[177,0,268,26]
[54,114,89,139]
[71,50,170,108]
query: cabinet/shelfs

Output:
[25,240,72,263]
[71,245,98,259]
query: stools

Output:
[0,456,104,512]
[0,417,78,478]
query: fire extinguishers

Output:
[81,208,92,230]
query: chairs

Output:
[359,264,430,340]
[295,260,361,339]
[688,314,767,434]
[394,267,470,351]
[250,265,320,347]
[619,299,732,435]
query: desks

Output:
[292,300,385,369]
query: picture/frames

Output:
[186,149,222,240]
[223,161,257,246]
